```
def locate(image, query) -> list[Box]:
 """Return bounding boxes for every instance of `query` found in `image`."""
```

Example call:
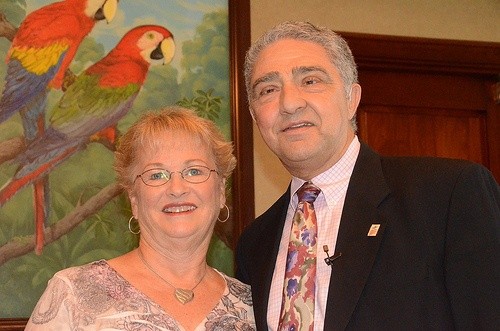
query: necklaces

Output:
[136,246,208,304]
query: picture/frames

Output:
[0,0,256,331]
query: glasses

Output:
[133,165,220,188]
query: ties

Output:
[276,179,324,331]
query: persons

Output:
[232,19,499,331]
[22,104,257,331]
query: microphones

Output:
[323,245,344,267]
